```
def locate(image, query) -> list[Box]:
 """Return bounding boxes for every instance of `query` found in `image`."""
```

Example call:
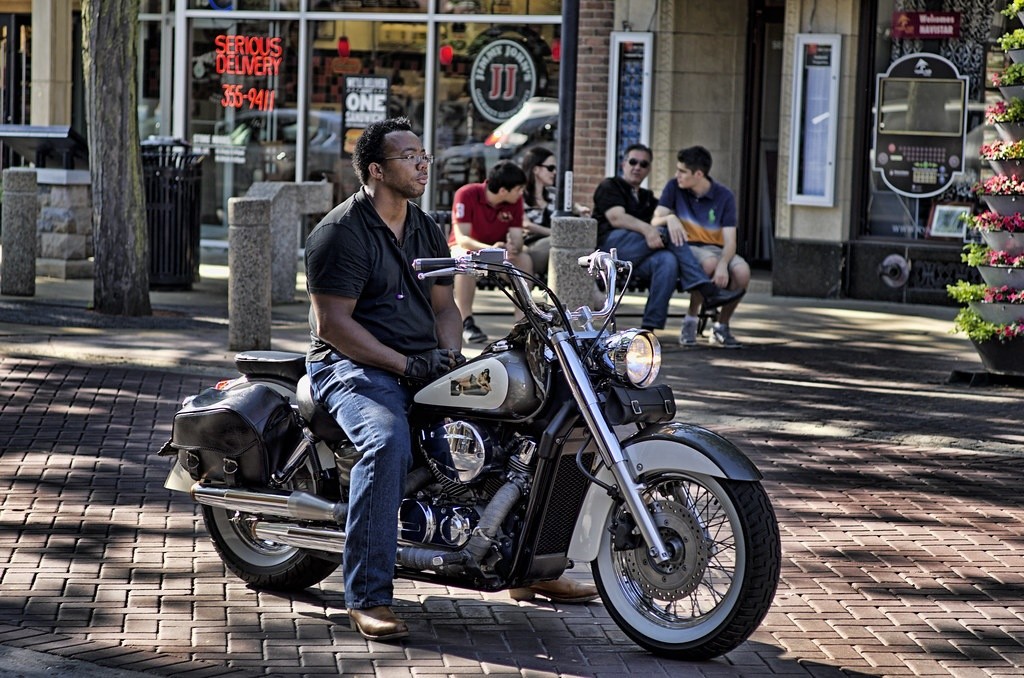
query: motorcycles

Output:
[158,247,782,661]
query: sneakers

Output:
[679,320,697,345]
[709,325,742,347]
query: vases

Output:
[967,9,1023,376]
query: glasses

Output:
[539,164,556,172]
[383,153,433,164]
[627,158,650,168]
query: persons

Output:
[591,143,746,338]
[650,146,752,348]
[303,116,600,640]
[448,145,590,346]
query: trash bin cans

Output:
[140,140,205,291]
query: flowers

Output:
[947,0,1024,345]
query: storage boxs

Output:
[174,385,297,488]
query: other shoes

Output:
[462,324,488,344]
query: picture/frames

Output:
[924,199,974,242]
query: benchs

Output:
[430,207,719,335]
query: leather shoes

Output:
[510,574,600,602]
[690,286,746,314]
[348,603,411,639]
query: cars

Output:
[437,96,560,180]
[811,98,1024,187]
[191,106,359,206]
